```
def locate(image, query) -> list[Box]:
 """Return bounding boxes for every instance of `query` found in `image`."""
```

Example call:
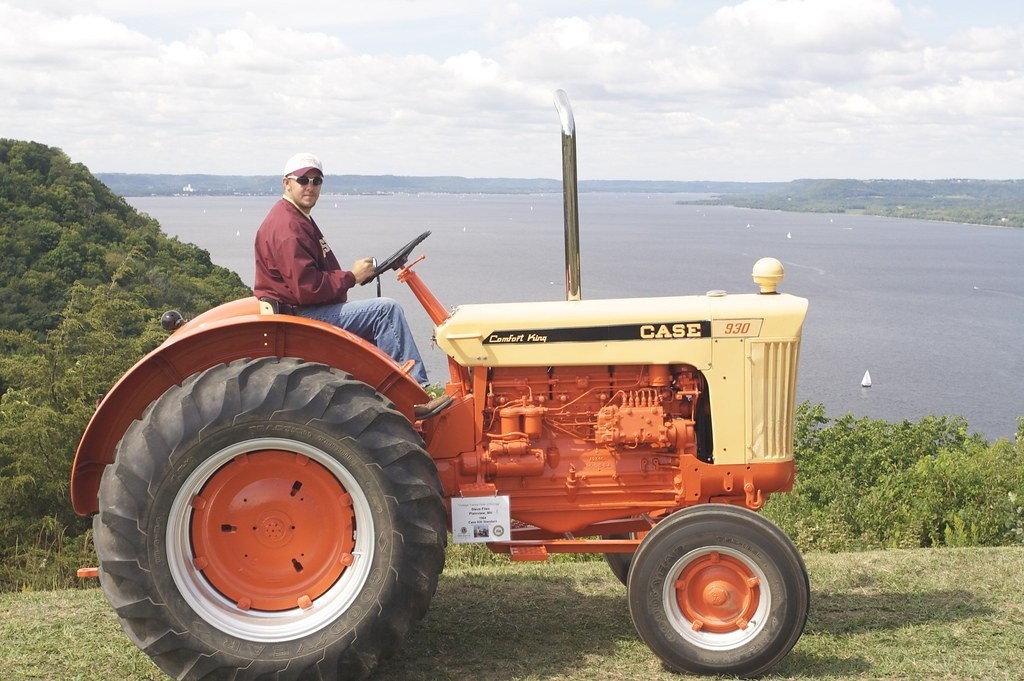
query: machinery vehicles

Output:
[71,88,812,680]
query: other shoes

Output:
[412,395,453,421]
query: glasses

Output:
[286,176,324,186]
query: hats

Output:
[285,153,324,178]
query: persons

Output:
[254,153,455,421]
